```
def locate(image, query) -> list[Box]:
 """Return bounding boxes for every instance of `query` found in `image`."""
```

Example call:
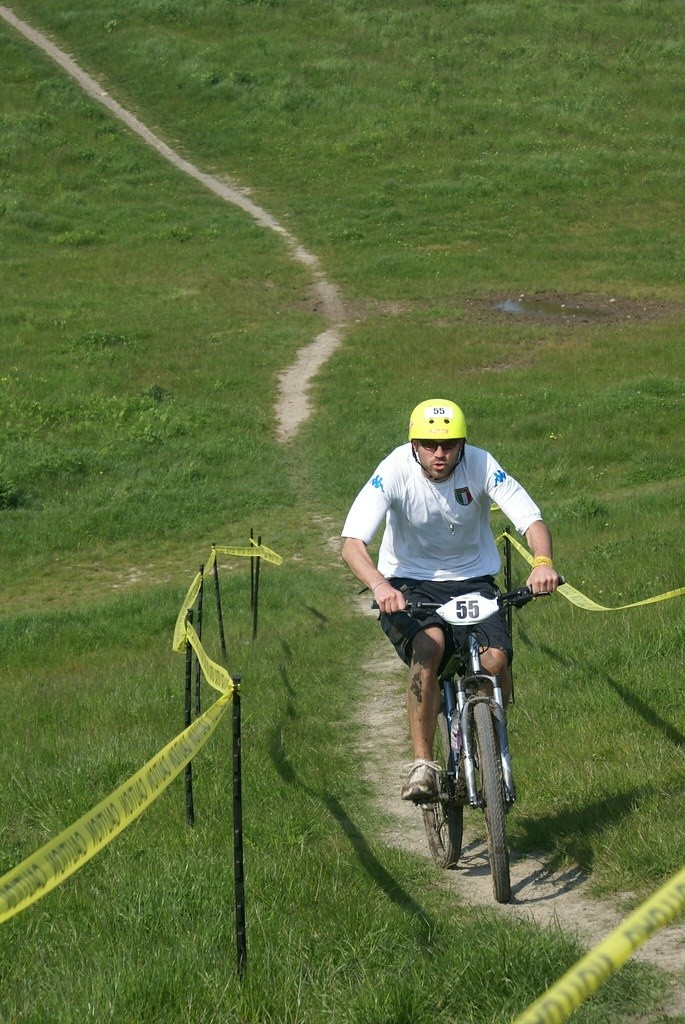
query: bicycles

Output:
[371,571,574,894]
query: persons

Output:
[343,398,558,801]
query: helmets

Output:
[408,399,467,442]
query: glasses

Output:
[417,440,460,451]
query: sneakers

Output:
[473,708,507,770]
[401,759,442,800]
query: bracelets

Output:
[533,556,552,568]
[373,581,389,597]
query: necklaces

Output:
[423,475,456,536]
